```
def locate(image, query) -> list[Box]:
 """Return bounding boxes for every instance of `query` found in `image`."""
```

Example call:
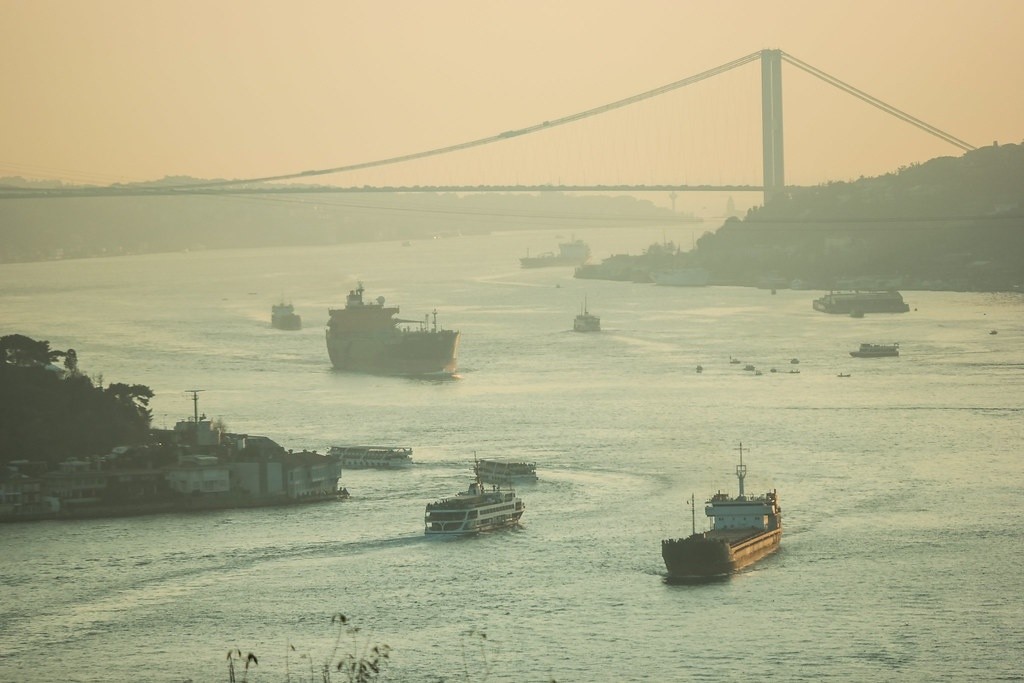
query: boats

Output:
[473,451,540,486]
[325,281,460,375]
[520,236,592,266]
[423,449,526,534]
[849,342,901,358]
[661,442,784,579]
[326,445,414,469]
[811,278,910,316]
[271,300,302,331]
[573,299,601,334]
